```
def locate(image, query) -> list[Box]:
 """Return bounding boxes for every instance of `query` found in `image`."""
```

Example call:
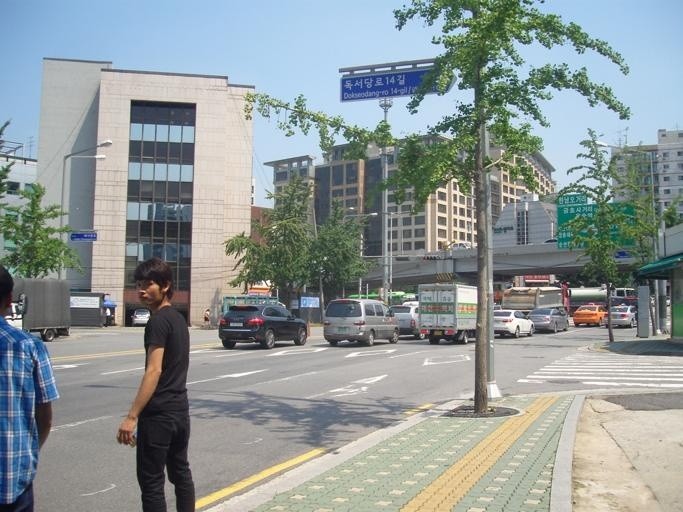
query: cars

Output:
[649,294,672,305]
[130,308,149,325]
[388,300,637,340]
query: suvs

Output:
[218,304,308,350]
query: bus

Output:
[350,290,418,309]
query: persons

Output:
[103,307,111,326]
[117,259,195,511]
[0,263,59,512]
[203,309,210,326]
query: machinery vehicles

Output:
[567,285,639,317]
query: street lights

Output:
[336,205,417,302]
[57,140,111,278]
[590,140,662,337]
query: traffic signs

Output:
[555,192,589,206]
[71,232,97,242]
[556,201,640,249]
[340,64,457,102]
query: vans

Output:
[323,298,401,346]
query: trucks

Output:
[5,274,74,343]
[416,284,481,343]
[501,281,572,318]
